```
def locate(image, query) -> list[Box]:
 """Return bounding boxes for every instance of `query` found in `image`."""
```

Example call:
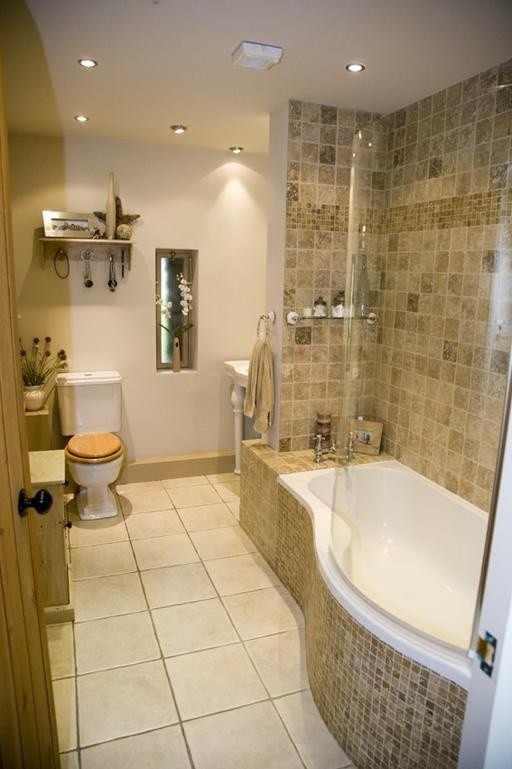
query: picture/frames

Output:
[42,210,97,238]
[349,420,383,456]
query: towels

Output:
[243,339,275,434]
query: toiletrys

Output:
[332,290,346,317]
[313,297,327,317]
[303,307,313,317]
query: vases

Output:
[173,337,181,372]
[24,384,45,411]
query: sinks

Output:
[224,360,250,388]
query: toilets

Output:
[55,371,126,522]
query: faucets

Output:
[346,432,357,464]
[312,435,335,462]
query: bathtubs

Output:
[277,460,490,691]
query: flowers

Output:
[18,336,66,386]
[157,273,193,337]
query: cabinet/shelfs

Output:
[28,449,75,624]
